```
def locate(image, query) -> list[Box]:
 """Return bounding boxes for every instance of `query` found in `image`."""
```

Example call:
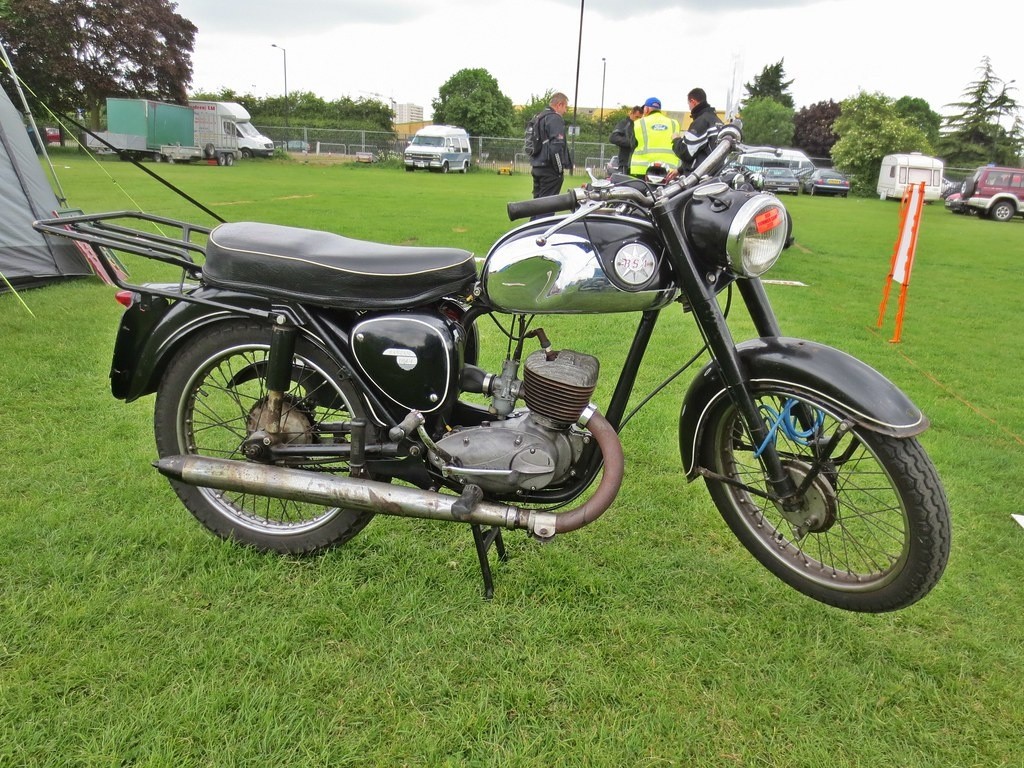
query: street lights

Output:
[598,57,607,142]
[271,44,288,139]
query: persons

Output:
[523,92,574,222]
[610,105,644,177]
[627,96,684,181]
[670,88,727,182]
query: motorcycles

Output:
[30,118,952,613]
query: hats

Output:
[641,97,661,110]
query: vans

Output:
[404,124,472,174]
[157,98,275,159]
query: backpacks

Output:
[524,112,561,156]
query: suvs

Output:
[960,166,1024,221]
[276,141,313,154]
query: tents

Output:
[0,42,229,319]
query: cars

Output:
[762,167,800,196]
[802,168,850,198]
